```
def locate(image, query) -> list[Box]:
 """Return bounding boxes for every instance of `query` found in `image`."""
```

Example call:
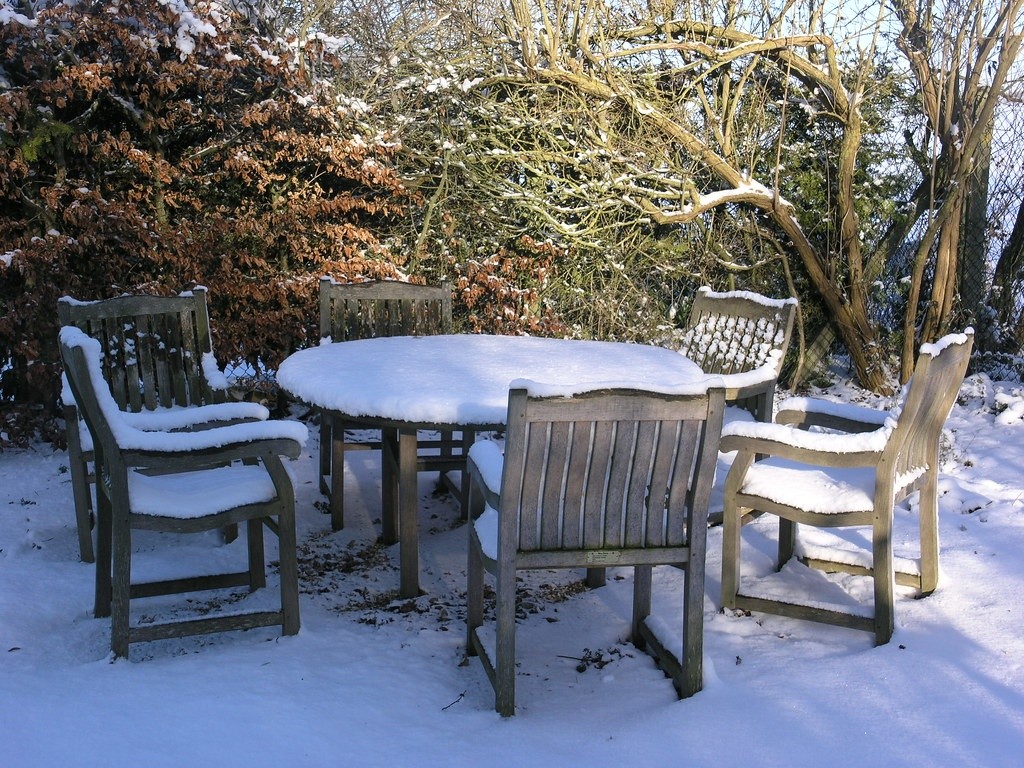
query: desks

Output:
[275,335,705,599]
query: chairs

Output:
[678,290,796,528]
[56,288,238,563]
[466,386,726,717]
[718,333,975,648]
[56,333,302,663]
[318,278,475,532]
